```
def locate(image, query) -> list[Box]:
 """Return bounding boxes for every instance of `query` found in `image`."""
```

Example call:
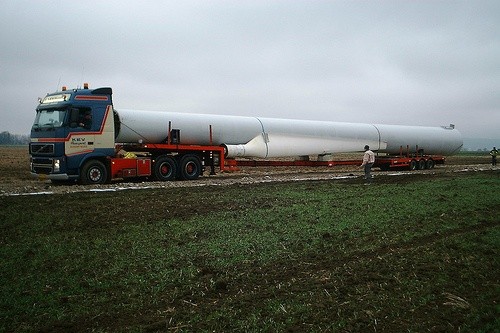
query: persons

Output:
[359,145,375,186]
[79,111,92,130]
[489,146,498,166]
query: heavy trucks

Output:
[29,82,453,187]
